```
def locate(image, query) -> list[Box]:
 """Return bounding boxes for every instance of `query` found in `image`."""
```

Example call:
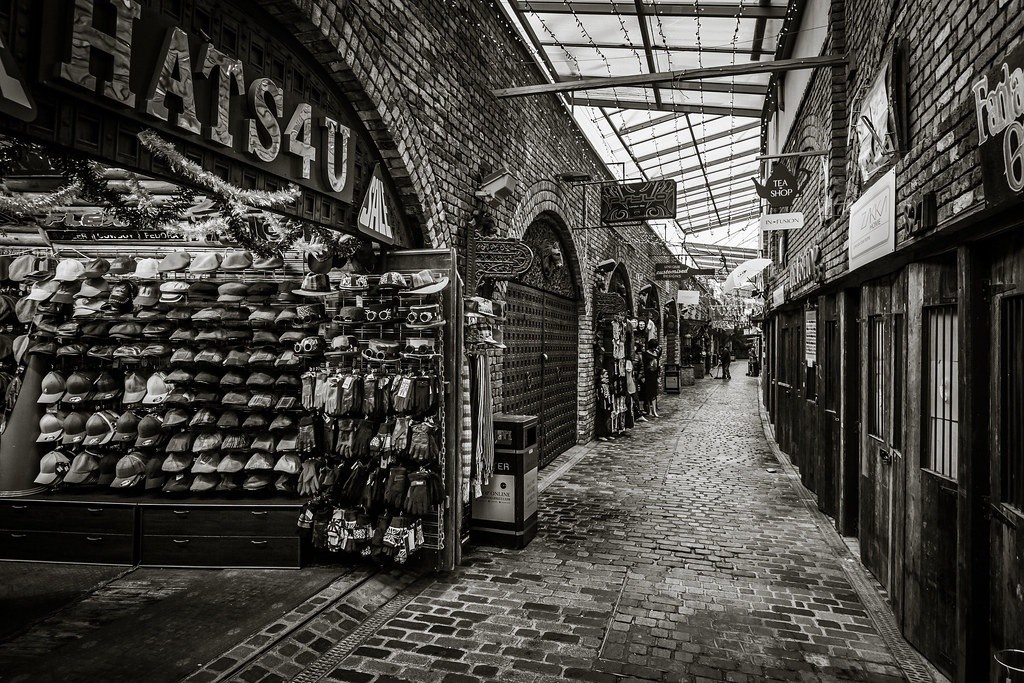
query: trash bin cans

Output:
[748,357,759,377]
[471,413,538,551]
[731,354,736,362]
[663,363,681,394]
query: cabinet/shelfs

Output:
[0,493,307,570]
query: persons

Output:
[596,366,615,440]
[625,359,648,422]
[644,339,660,418]
[717,344,731,379]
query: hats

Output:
[398,337,443,358]
[332,306,366,325]
[291,269,449,297]
[361,338,402,361]
[363,302,401,325]
[324,335,361,356]
[294,336,327,356]
[465,297,497,318]
[23,250,304,492]
[406,304,446,329]
[0,255,43,398]
[291,303,330,329]
[464,322,507,349]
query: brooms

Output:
[713,358,722,379]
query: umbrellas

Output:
[723,258,774,301]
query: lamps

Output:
[596,258,617,272]
[474,168,517,208]
[554,172,591,182]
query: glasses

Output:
[294,308,438,361]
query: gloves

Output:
[297,459,442,514]
[297,500,425,565]
[296,416,440,461]
[301,368,436,415]
